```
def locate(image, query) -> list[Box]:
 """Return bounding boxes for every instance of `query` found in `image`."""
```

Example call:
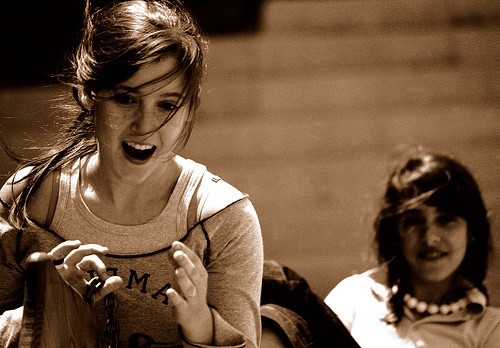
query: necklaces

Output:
[384,273,470,316]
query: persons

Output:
[321,152,500,348]
[0,0,263,348]
